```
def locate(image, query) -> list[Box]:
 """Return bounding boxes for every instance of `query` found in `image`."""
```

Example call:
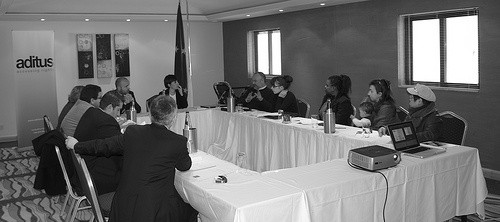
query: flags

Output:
[174,0,189,110]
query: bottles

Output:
[229,89,233,98]
[185,111,192,129]
[326,100,332,113]
[130,101,135,110]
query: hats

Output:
[407,83,436,102]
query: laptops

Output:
[386,119,448,159]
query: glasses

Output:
[272,84,278,88]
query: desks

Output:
[112,102,489,222]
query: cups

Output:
[183,128,197,154]
[323,112,335,134]
[126,110,137,123]
[238,104,243,112]
[311,115,318,129]
[363,127,370,138]
[227,97,235,112]
[278,110,284,121]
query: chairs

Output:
[436,109,469,148]
[297,98,311,119]
[42,115,105,222]
[145,94,158,113]
[212,81,236,105]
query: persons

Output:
[100,77,141,114]
[64,95,198,222]
[159,74,188,109]
[318,74,354,127]
[238,72,298,115]
[74,95,125,196]
[59,84,103,138]
[350,78,395,132]
[378,83,447,143]
[58,86,85,128]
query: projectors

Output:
[348,145,402,171]
